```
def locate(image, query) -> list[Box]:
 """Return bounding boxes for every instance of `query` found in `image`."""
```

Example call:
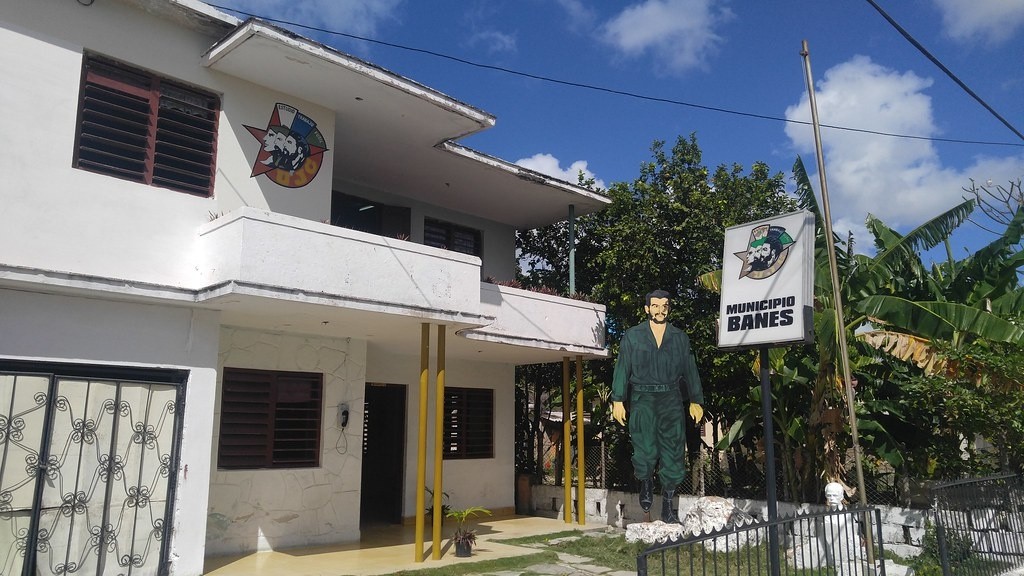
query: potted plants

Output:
[445,505,493,557]
[425,486,453,527]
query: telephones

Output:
[337,404,350,429]
